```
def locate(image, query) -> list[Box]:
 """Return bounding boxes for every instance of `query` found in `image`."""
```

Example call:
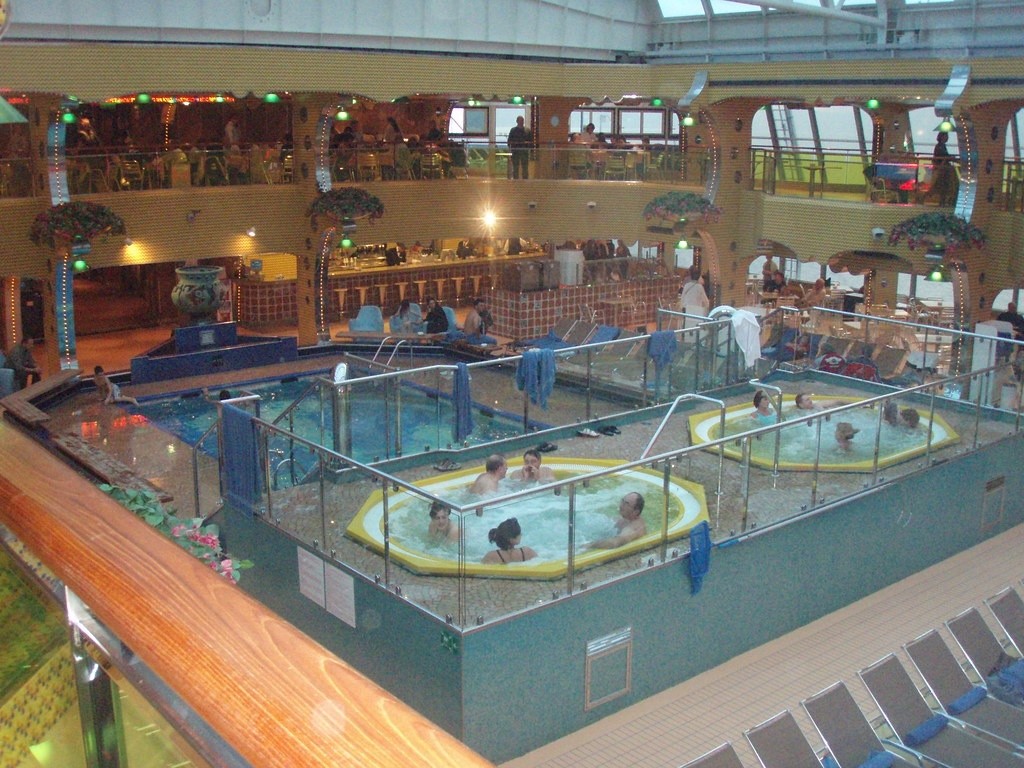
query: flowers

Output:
[29,199,125,244]
[303,184,383,225]
[888,210,986,251]
[643,190,724,227]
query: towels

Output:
[647,330,678,366]
[434,331,464,346]
[997,660,1024,699]
[451,362,474,447]
[587,325,620,344]
[856,750,895,768]
[516,348,556,412]
[685,519,710,599]
[762,326,823,362]
[902,714,948,746]
[731,308,761,371]
[466,334,497,346]
[948,686,987,715]
[505,330,578,352]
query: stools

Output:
[330,274,501,321]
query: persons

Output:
[94,366,139,407]
[585,492,648,550]
[751,391,783,425]
[996,302,1024,350]
[899,409,920,428]
[507,116,533,179]
[777,335,811,366]
[566,123,653,181]
[202,387,231,408]
[762,250,788,294]
[852,346,880,380]
[679,264,712,347]
[393,296,498,347]
[932,133,959,206]
[881,403,902,425]
[511,450,556,485]
[367,237,633,285]
[794,278,826,308]
[481,518,537,564]
[427,501,463,551]
[4,336,42,392]
[469,454,507,501]
[108,114,292,191]
[796,393,851,416]
[329,115,465,181]
[835,423,860,456]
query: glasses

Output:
[621,498,635,509]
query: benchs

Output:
[454,339,501,357]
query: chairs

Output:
[568,144,664,181]
[1,146,488,194]
[390,303,422,332]
[864,174,899,204]
[348,305,384,332]
[681,585,1024,768]
[745,279,954,386]
[513,295,725,394]
[422,307,456,333]
[0,350,25,396]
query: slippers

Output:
[434,458,461,472]
[598,426,614,436]
[608,425,621,434]
[578,428,600,437]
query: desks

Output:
[597,300,631,328]
[895,310,908,316]
[827,284,854,292]
[758,292,799,300]
[844,322,860,329]
[920,300,954,326]
[593,150,650,169]
[336,331,445,346]
[916,334,952,353]
[496,152,512,179]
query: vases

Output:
[922,233,947,242]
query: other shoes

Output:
[536,442,558,451]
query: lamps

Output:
[136,92,151,104]
[672,235,693,250]
[679,112,701,127]
[335,234,355,247]
[71,226,257,270]
[334,104,350,121]
[932,115,958,133]
[508,96,526,106]
[527,201,597,208]
[264,93,281,104]
[923,245,947,282]
[60,107,78,124]
[871,228,886,241]
